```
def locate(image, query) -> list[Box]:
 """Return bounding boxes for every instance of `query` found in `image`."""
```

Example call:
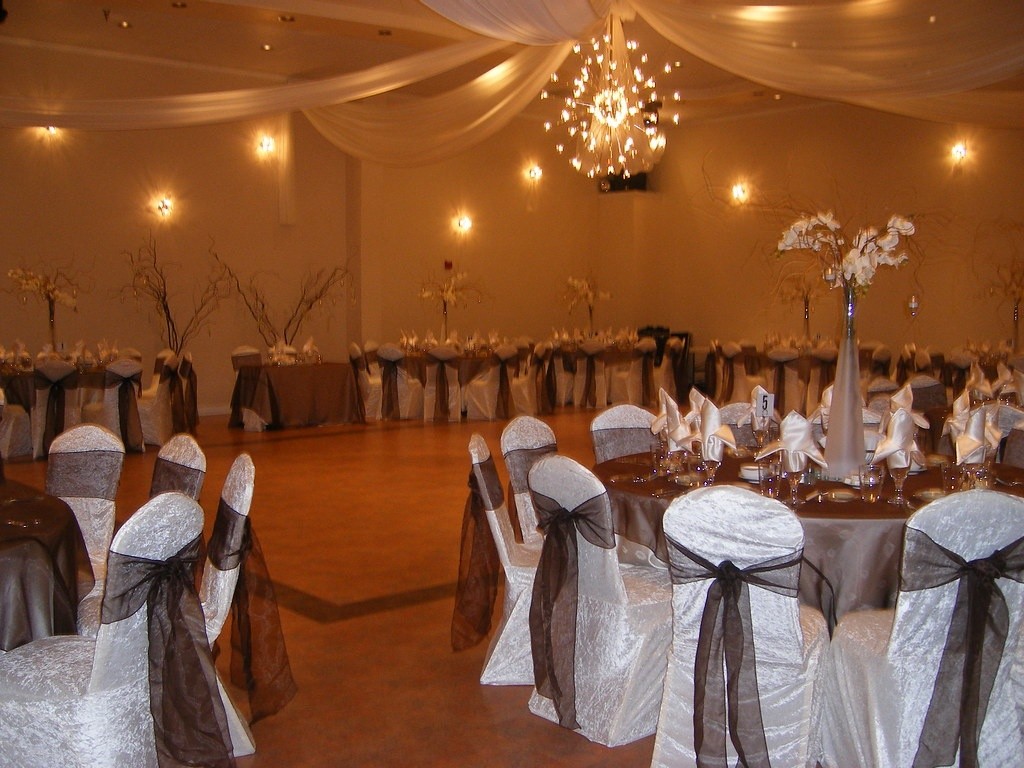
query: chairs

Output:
[0,331,1024,768]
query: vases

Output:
[812,289,867,479]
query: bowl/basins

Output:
[738,463,764,479]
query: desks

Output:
[222,363,362,428]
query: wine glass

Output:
[660,427,996,510]
[970,386,1024,412]
[0,336,1024,372]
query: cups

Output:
[940,462,967,497]
[760,466,782,497]
[860,465,886,503]
[687,454,705,480]
[650,443,666,470]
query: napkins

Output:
[870,405,928,468]
[941,388,972,446]
[684,385,709,430]
[650,382,682,442]
[954,403,988,467]
[681,394,738,463]
[984,404,1002,454]
[962,362,993,399]
[877,380,932,434]
[992,359,1017,397]
[752,408,829,472]
[737,384,775,432]
[662,398,695,454]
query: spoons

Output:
[996,477,1024,487]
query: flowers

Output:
[4,267,77,308]
[776,209,916,297]
[559,275,613,313]
[415,270,484,310]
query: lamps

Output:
[532,11,685,181]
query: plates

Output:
[738,473,759,484]
[822,488,859,503]
[674,475,704,486]
[913,487,946,502]
[925,454,950,467]
[729,484,760,494]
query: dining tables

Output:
[574,451,1024,616]
